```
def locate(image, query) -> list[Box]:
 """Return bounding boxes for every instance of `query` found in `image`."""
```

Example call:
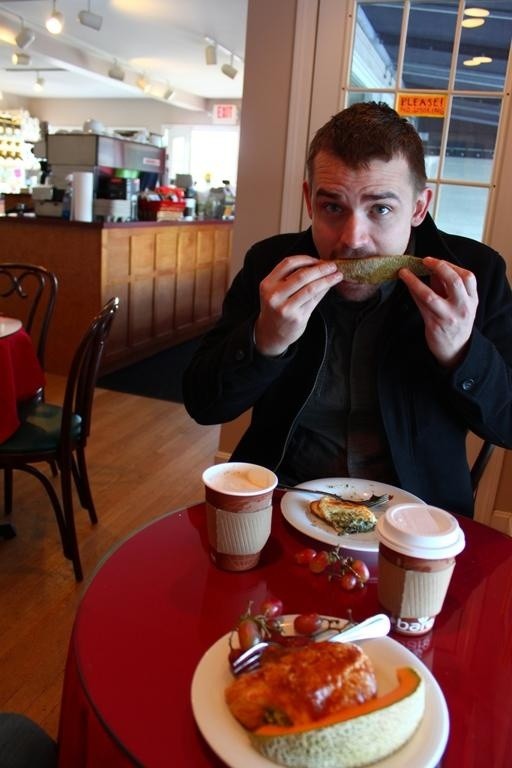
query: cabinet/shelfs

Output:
[0,117,25,193]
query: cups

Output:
[70,173,94,221]
[198,460,281,571]
[372,505,469,635]
[403,636,438,669]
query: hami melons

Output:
[320,254,435,286]
[251,666,427,767]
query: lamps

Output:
[13,16,36,50]
[11,53,33,66]
[222,52,238,79]
[45,0,65,35]
[204,37,217,64]
[79,0,102,31]
[107,57,125,81]
[35,70,45,86]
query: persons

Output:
[179,99,512,525]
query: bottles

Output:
[183,197,198,221]
[220,179,235,222]
[0,112,23,164]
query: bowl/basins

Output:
[52,118,110,136]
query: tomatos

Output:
[229,546,370,670]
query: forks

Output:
[280,482,397,512]
[230,613,391,676]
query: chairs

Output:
[0,263,58,414]
[0,714,60,768]
[1,294,119,582]
[461,425,496,521]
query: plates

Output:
[95,199,131,217]
[280,478,428,553]
[31,184,53,200]
[114,130,139,136]
[189,612,450,768]
